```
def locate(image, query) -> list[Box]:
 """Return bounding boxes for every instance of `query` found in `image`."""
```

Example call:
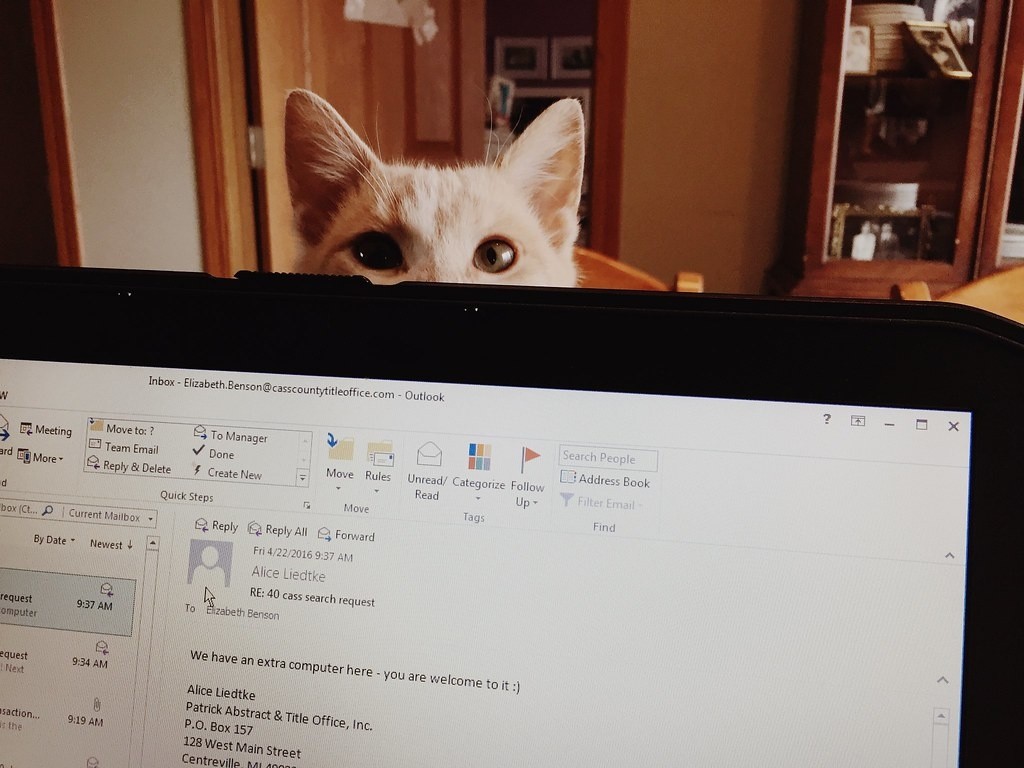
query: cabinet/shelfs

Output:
[786,0,1024,297]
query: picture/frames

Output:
[494,36,547,79]
[509,87,590,142]
[550,37,594,79]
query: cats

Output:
[283,87,587,290]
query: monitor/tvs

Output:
[0,264,1024,768]
